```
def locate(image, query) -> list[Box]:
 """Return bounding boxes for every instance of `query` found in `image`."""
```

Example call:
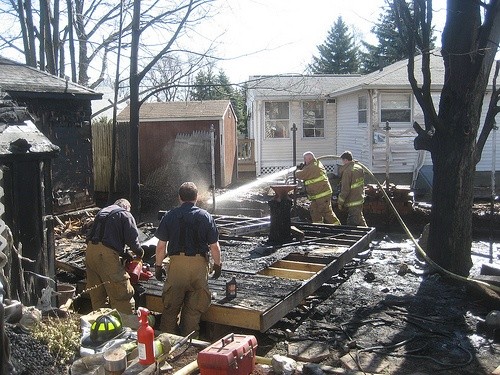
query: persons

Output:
[295,151,368,227]
[85,198,145,315]
[154,181,221,339]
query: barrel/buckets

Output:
[57,284,76,308]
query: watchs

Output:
[153,263,163,268]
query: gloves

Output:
[155,263,167,282]
[209,262,222,280]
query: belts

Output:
[86,240,120,252]
[169,251,206,257]
